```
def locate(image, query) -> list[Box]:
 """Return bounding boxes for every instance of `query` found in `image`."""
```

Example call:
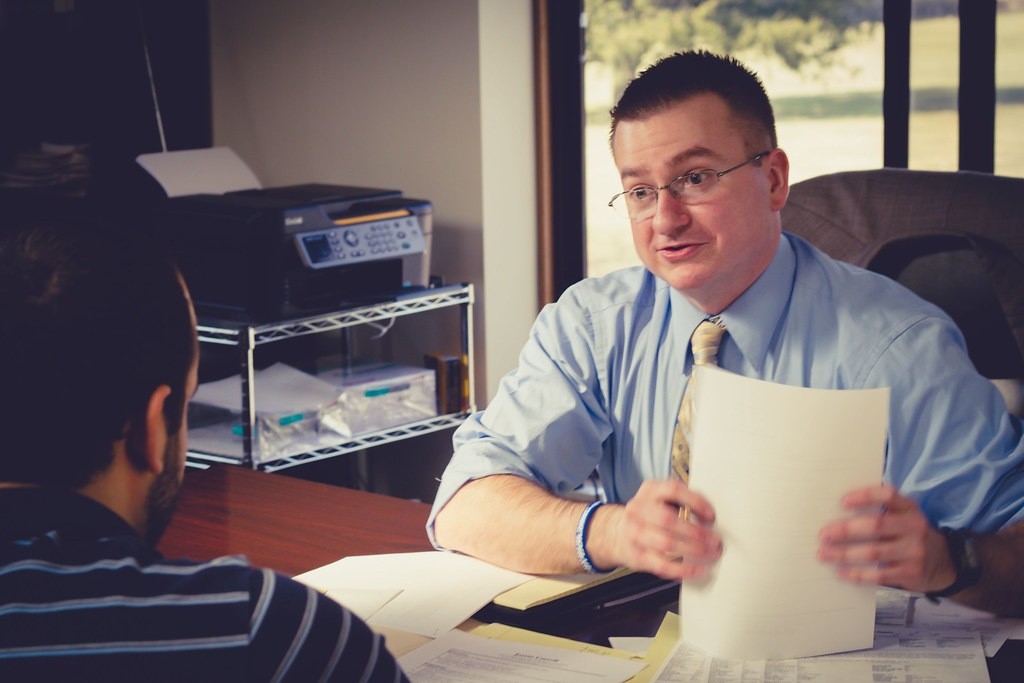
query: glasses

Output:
[608,151,769,220]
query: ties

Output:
[671,322,725,522]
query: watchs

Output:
[920,523,982,607]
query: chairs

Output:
[780,167,1024,430]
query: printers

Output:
[144,182,433,343]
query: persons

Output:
[425,49,1024,623]
[0,220,411,683]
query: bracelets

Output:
[575,500,619,576]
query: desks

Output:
[151,463,435,575]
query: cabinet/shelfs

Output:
[185,281,478,493]
